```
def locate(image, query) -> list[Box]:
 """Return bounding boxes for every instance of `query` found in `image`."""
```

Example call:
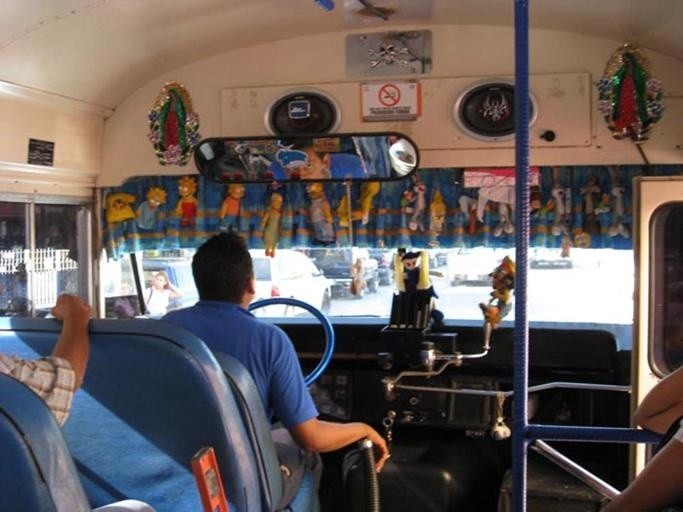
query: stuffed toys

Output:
[104,173,632,328]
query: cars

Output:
[450,246,498,286]
[528,248,575,270]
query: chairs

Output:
[213,353,314,512]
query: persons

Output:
[0,294,96,429]
[268,139,366,179]
[105,173,632,329]
[162,230,393,511]
[601,366,682,511]
